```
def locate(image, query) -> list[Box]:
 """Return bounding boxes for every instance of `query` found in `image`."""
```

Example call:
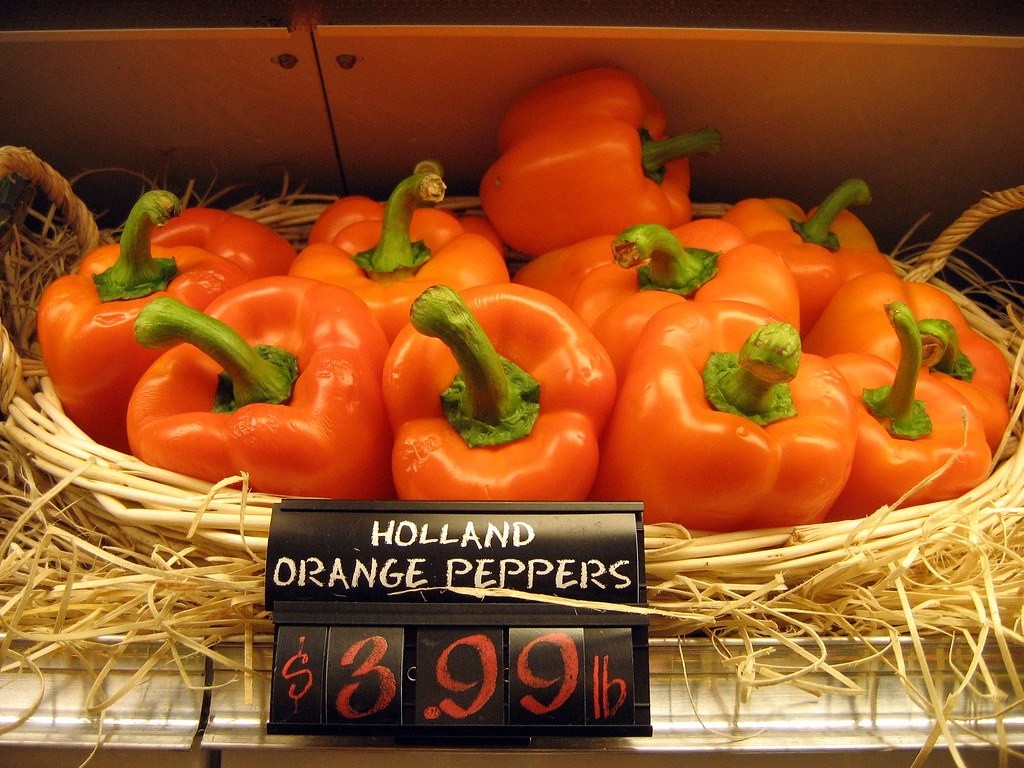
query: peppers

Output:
[39,65,1014,534]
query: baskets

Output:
[0,147,1024,639]
